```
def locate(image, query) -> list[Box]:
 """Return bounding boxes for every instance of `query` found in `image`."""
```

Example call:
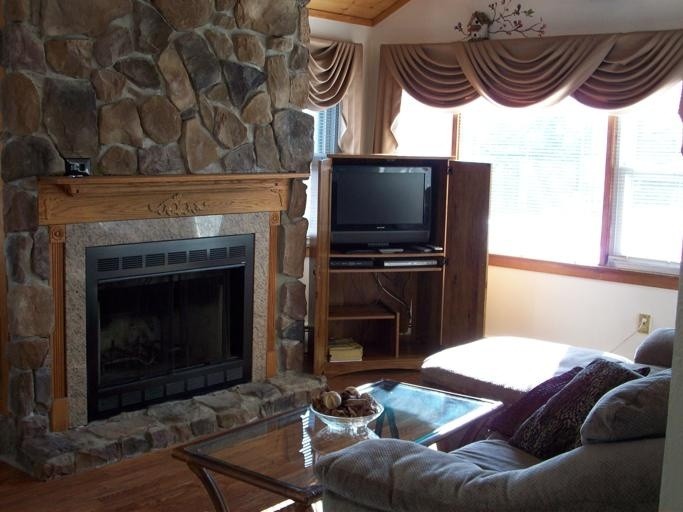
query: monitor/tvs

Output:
[331,166,433,252]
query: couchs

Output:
[314,431,665,511]
[420,327,675,452]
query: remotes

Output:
[427,243,444,253]
[405,245,433,252]
[378,248,404,253]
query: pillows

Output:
[486,358,672,459]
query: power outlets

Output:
[638,314,650,334]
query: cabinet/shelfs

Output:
[309,154,490,378]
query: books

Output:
[327,336,364,363]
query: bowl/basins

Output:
[309,401,385,430]
